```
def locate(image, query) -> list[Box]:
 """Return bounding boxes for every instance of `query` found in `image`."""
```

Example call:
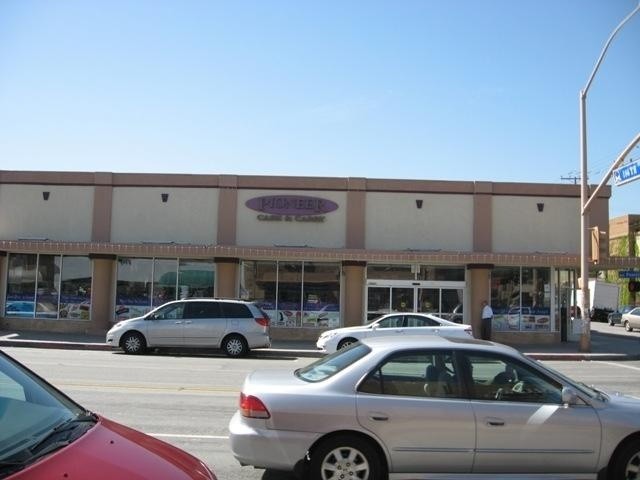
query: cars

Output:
[5,300,91,322]
[506,306,531,329]
[315,310,477,357]
[223,336,640,478]
[620,306,640,333]
[0,344,218,479]
[257,301,340,325]
[608,305,636,326]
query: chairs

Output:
[424,366,452,398]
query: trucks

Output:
[572,279,620,323]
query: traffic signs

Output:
[612,158,640,187]
[619,271,640,278]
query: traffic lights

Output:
[628,281,640,292]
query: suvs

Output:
[451,304,463,324]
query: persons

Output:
[465,360,506,401]
[480,300,493,340]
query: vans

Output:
[104,298,271,356]
